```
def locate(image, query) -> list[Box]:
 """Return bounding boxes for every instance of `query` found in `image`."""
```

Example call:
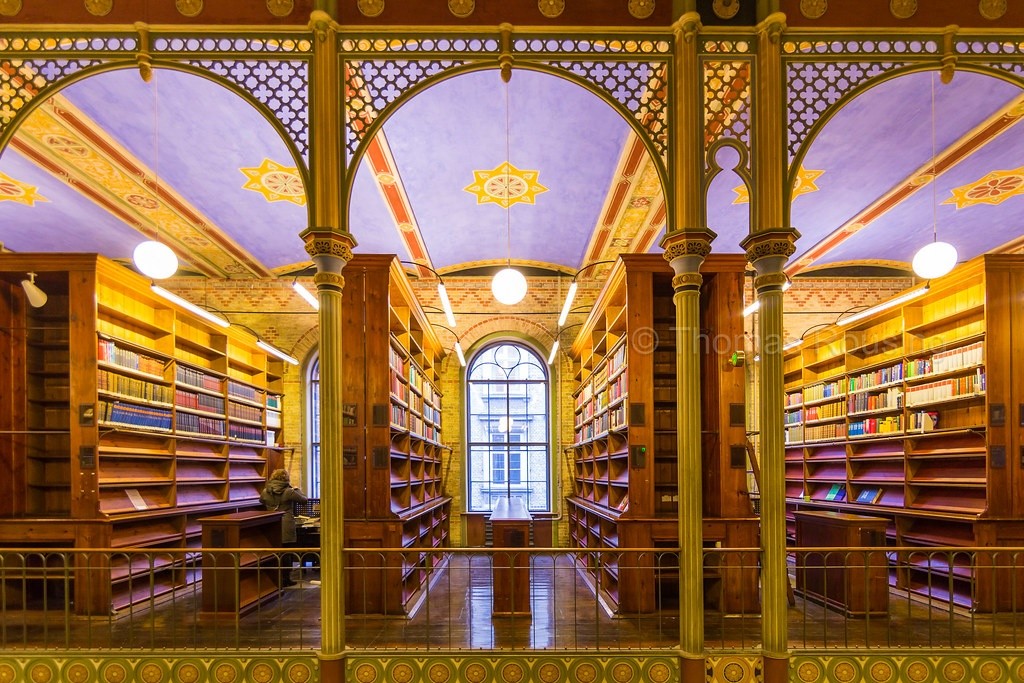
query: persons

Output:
[259,469,308,587]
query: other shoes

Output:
[281,578,297,588]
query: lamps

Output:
[558,261,615,326]
[21,272,48,309]
[548,323,585,365]
[491,82,527,306]
[400,259,456,328]
[151,283,231,329]
[132,70,179,280]
[753,322,831,363]
[912,73,957,280]
[228,322,300,366]
[292,263,319,311]
[833,259,968,326]
[430,324,466,367]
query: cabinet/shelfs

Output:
[0,252,285,620]
[783,253,1024,619]
[566,252,762,620]
[316,253,454,621]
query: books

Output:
[825,483,883,504]
[389,345,442,445]
[785,340,986,444]
[573,341,629,445]
[98,337,283,446]
[294,515,321,529]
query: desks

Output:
[196,510,285,619]
[489,495,534,618]
[791,511,893,619]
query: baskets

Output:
[293,499,321,518]
